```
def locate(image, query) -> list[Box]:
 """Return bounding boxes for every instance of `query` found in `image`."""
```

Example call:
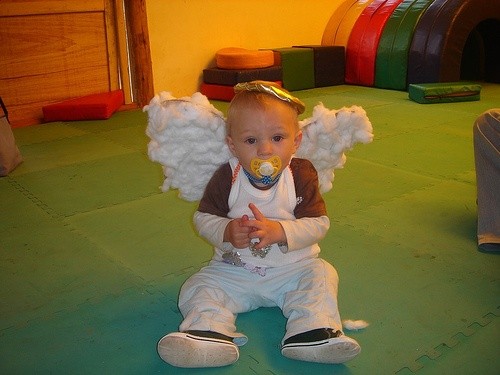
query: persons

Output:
[0,95,25,179]
[140,77,375,368]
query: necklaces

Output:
[242,168,282,185]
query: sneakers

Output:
[281,329,361,364]
[157,330,240,368]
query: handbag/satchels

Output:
[0,97,23,177]
